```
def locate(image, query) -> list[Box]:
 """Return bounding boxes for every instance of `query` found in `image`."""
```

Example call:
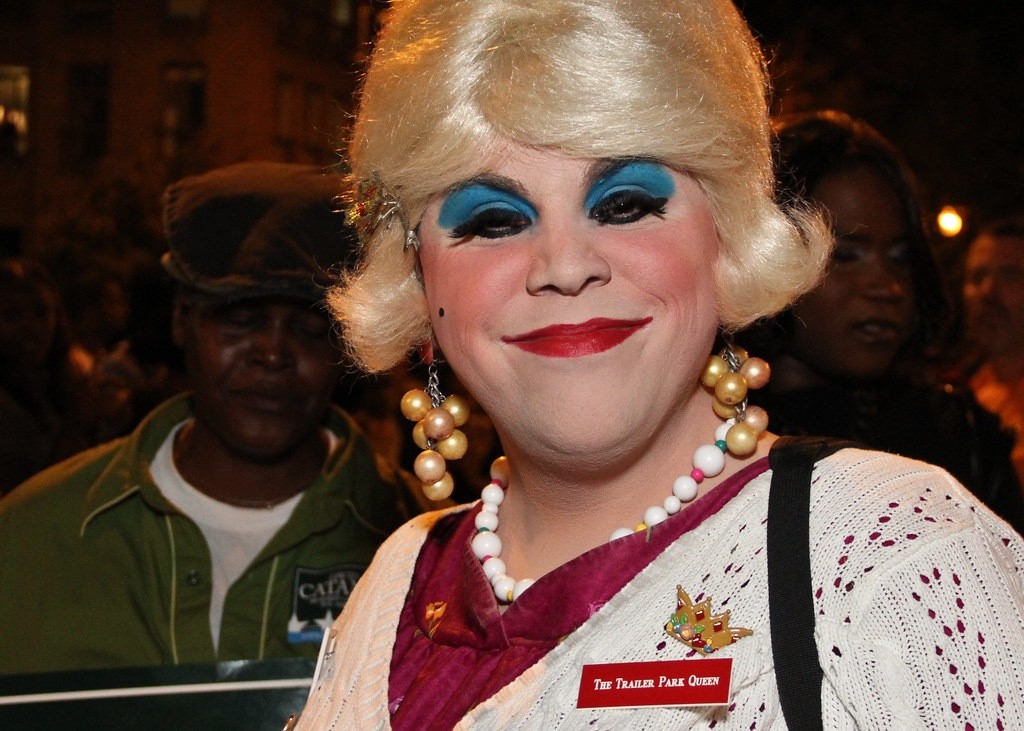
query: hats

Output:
[160,160,359,307]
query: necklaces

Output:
[470,411,740,609]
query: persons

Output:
[0,174,193,488]
[727,103,1023,537]
[959,220,1024,464]
[285,0,1024,731]
[0,161,459,676]
